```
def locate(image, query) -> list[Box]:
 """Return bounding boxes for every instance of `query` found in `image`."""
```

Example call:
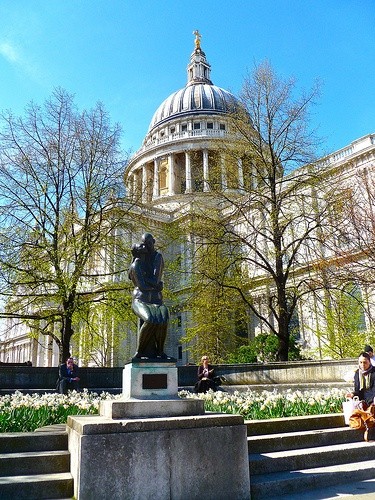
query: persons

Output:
[128,243,164,362]
[56,357,83,394]
[193,355,222,393]
[139,233,177,361]
[345,351,375,442]
[364,344,375,366]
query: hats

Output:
[364,346,372,352]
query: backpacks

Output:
[349,402,375,429]
